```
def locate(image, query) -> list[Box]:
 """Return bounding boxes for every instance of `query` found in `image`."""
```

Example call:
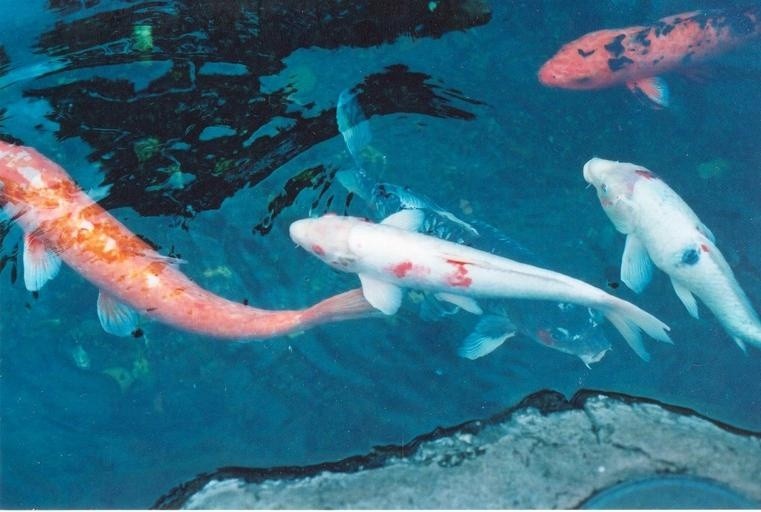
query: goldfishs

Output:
[326,89,614,366]
